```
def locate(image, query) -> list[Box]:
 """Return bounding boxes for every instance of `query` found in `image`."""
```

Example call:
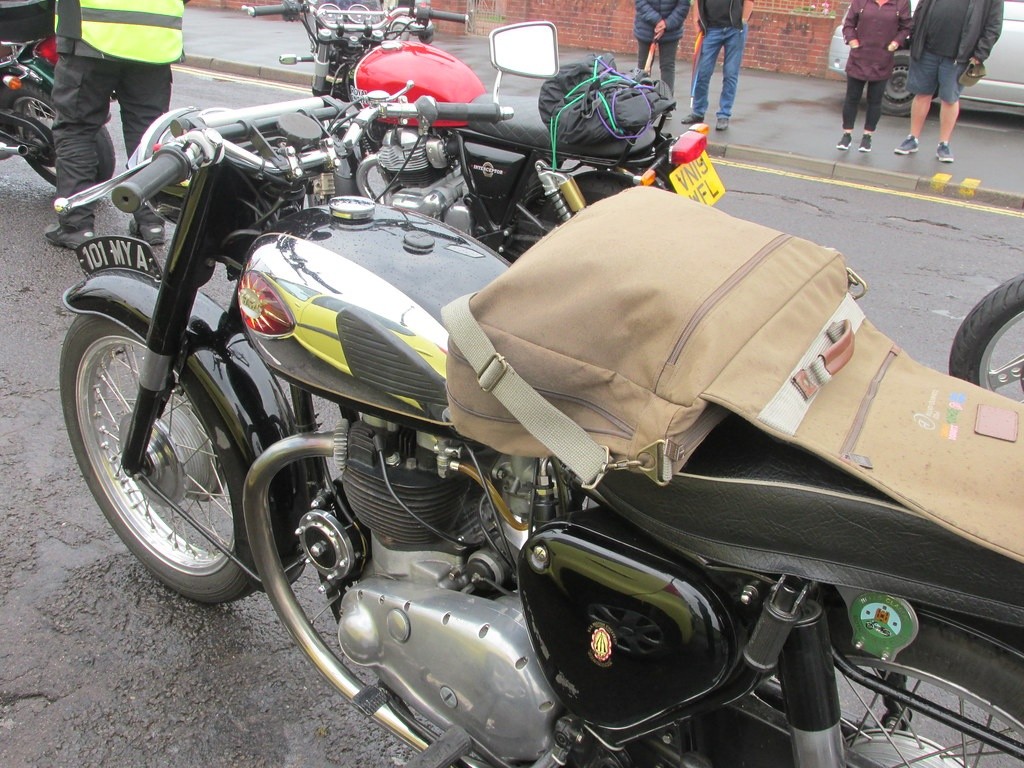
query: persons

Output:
[43,0,184,250]
[632,0,690,120]
[835,0,912,153]
[680,0,754,131]
[894,0,1004,162]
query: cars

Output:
[827,0,1024,118]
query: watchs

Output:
[891,42,898,50]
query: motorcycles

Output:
[242,0,729,263]
[0,31,115,189]
[53,80,1023,768]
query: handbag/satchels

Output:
[439,186,1024,564]
[538,54,675,146]
[958,61,987,87]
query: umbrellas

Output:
[642,39,657,78]
[689,30,704,109]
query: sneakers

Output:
[44,223,96,249]
[858,134,872,152]
[837,132,852,149]
[893,134,919,154]
[936,142,955,161]
[130,218,167,243]
[716,119,728,129]
[681,113,704,122]
